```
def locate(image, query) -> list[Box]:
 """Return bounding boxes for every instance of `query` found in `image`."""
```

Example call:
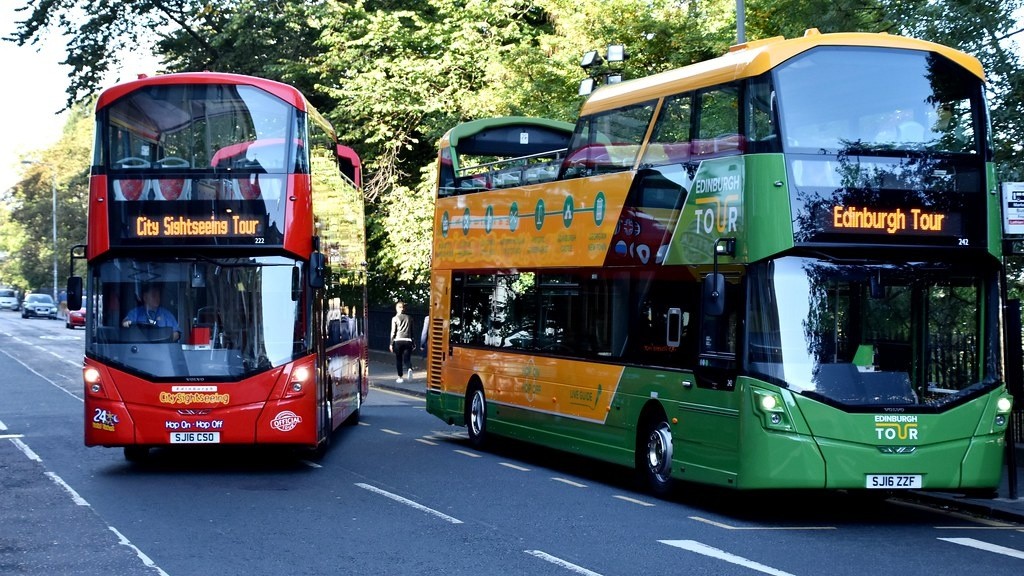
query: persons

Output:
[420,316,429,351]
[326,299,361,344]
[122,285,183,342]
[389,302,416,383]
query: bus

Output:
[425,28,1015,512]
[66,71,370,465]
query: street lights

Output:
[21,160,59,304]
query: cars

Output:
[20,293,58,321]
[0,290,19,311]
[64,294,100,329]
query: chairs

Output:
[436,133,794,197]
[113,157,293,200]
[897,122,925,142]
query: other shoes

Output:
[396,378,404,383]
[407,368,413,383]
